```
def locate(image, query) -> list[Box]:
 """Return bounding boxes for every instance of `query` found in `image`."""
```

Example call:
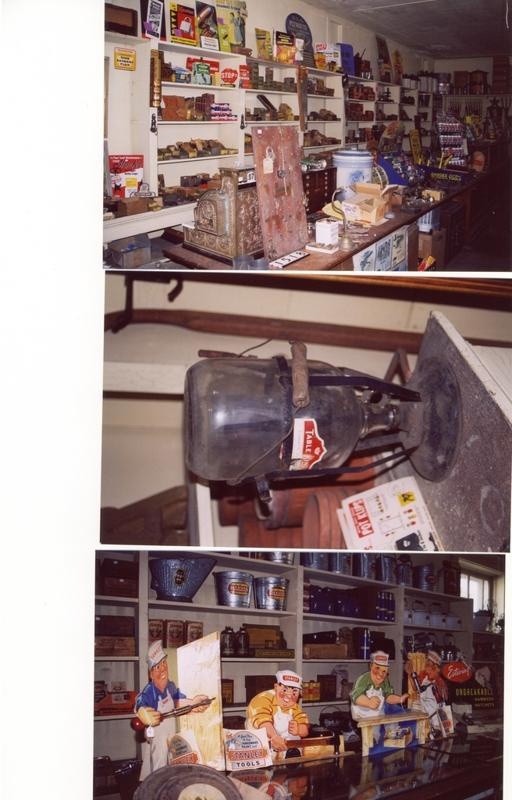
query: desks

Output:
[221,730,503,799]
[162,184,471,271]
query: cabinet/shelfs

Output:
[156,42,243,196]
[304,67,344,166]
[94,550,143,798]
[105,33,155,197]
[244,56,303,187]
[376,82,398,151]
[437,122,465,159]
[344,74,376,151]
[302,168,337,223]
[301,568,403,725]
[144,552,300,726]
[416,91,433,151]
[444,96,485,117]
[403,587,474,725]
[474,633,504,724]
[399,86,416,151]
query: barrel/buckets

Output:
[331,149,375,201]
[253,575,290,612]
[212,569,255,609]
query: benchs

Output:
[357,712,428,756]
